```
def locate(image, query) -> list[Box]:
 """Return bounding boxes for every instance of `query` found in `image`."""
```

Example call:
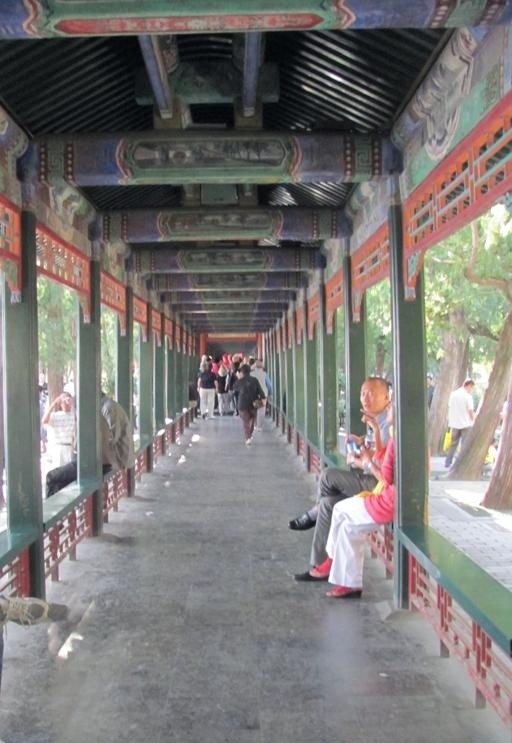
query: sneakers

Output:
[255,427,258,430]
[246,440,252,446]
[250,437,254,442]
[259,428,262,432]
[202,413,238,418]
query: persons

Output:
[42,391,75,467]
[46,398,112,499]
[310,388,396,598]
[100,387,133,469]
[288,374,390,581]
[446,376,475,466]
[196,346,273,443]
[426,373,435,408]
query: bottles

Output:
[347,441,359,469]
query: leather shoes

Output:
[295,571,330,581]
[288,512,316,529]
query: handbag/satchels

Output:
[442,433,451,451]
[253,399,263,409]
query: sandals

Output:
[327,587,361,598]
[309,559,331,576]
